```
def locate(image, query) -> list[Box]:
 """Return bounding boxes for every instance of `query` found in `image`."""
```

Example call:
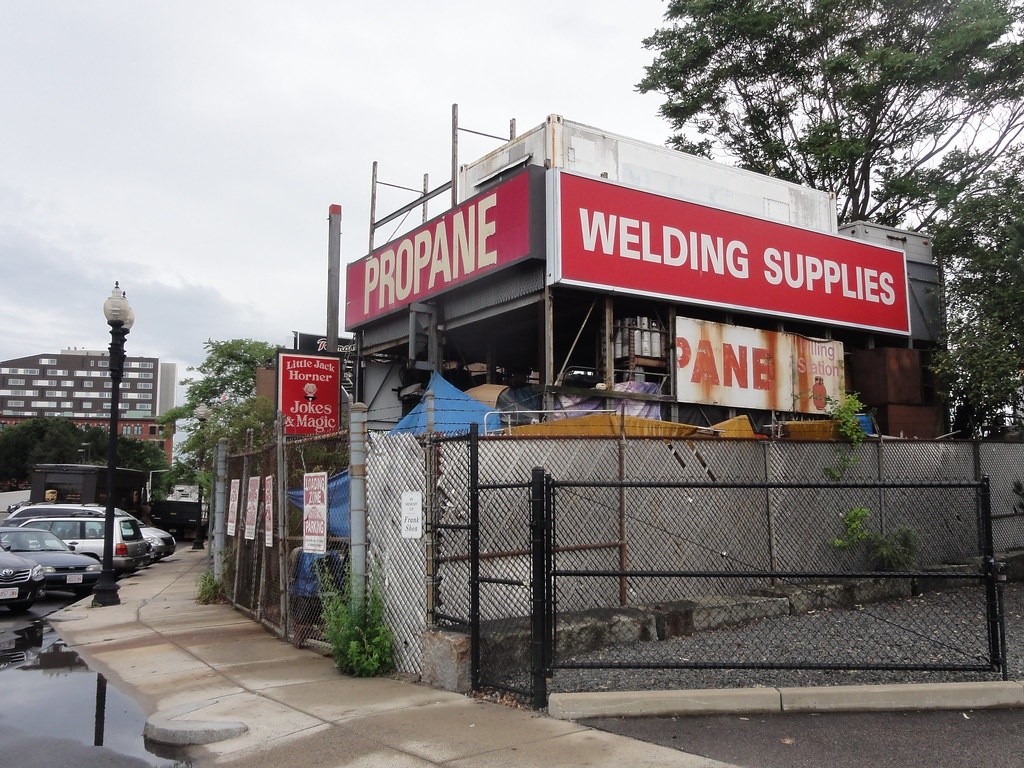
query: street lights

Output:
[90,279,136,606]
[191,403,209,550]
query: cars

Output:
[0,546,47,613]
[0,526,101,598]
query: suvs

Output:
[13,512,146,578]
[10,502,176,567]
